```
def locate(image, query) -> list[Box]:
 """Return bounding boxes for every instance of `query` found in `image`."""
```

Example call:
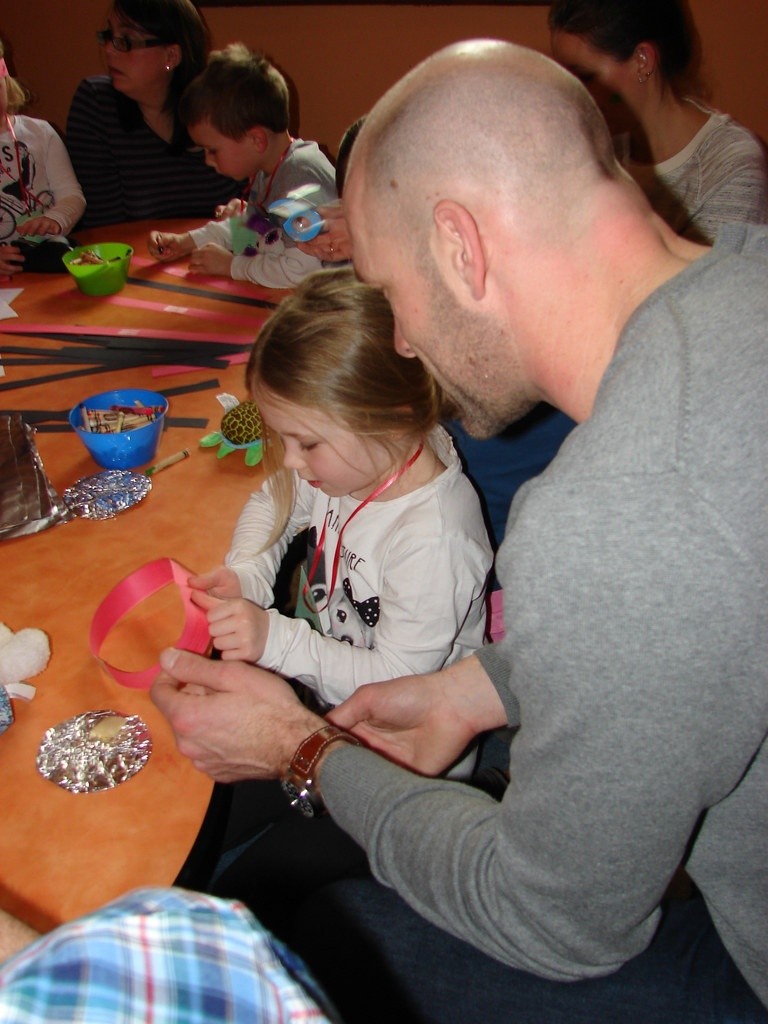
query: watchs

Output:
[280,724,365,821]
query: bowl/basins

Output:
[62,242,131,298]
[68,387,168,470]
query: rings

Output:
[329,244,334,255]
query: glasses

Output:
[96,31,170,52]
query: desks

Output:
[0,216,340,943]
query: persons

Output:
[147,37,767,1024]
[2,879,338,1024]
[67,1,255,234]
[0,40,87,280]
[294,2,766,652]
[146,43,349,291]
[187,264,497,910]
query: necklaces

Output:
[302,438,425,614]
